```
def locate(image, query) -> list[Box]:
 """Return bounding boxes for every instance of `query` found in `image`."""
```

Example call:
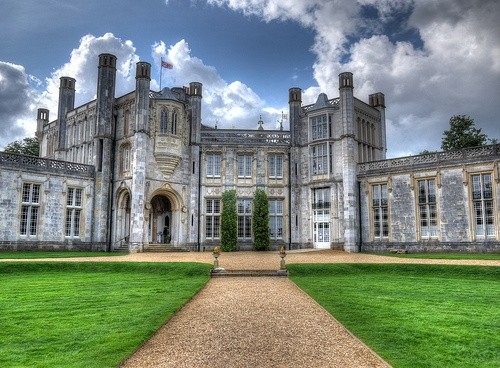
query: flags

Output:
[161,61,173,69]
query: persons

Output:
[163,226,169,241]
